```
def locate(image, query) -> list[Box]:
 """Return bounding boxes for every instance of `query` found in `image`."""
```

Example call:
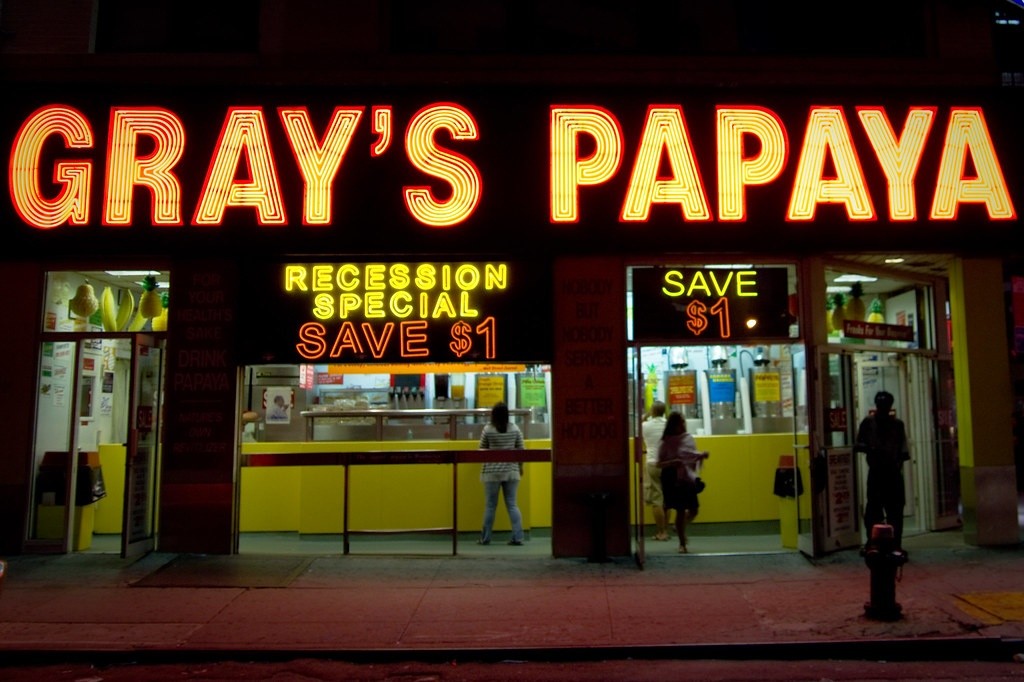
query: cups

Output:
[444,432,449,438]
[468,432,473,440]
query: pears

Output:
[71,284,98,318]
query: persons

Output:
[477,400,525,546]
[852,389,911,560]
[642,401,672,542]
[657,411,710,554]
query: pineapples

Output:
[826,296,834,333]
[832,293,846,330]
[868,297,884,323]
[847,282,866,321]
[140,276,162,317]
[152,291,169,331]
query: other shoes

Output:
[507,538,525,545]
[679,544,687,553]
[476,537,490,545]
[672,524,676,531]
[652,533,672,541]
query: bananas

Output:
[100,287,116,332]
[129,294,146,332]
[116,289,134,332]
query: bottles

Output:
[407,429,413,439]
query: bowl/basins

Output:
[311,400,387,425]
